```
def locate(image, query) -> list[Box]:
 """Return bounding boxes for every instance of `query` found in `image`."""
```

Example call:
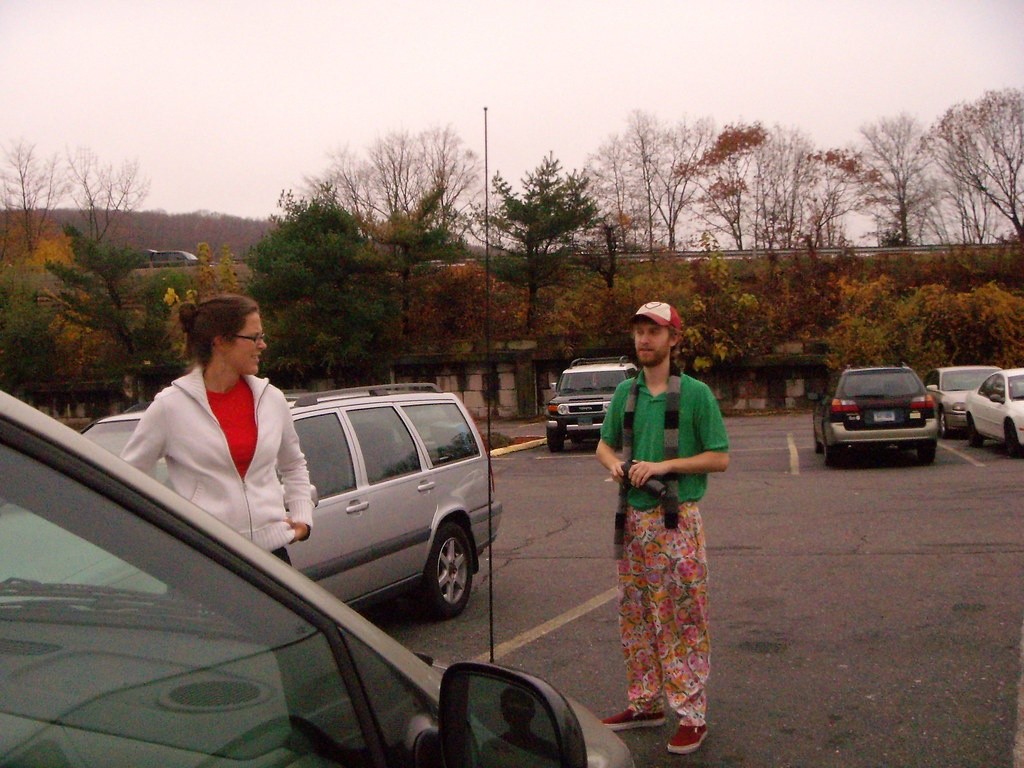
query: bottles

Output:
[622,462,667,496]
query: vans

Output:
[151,250,200,266]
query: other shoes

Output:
[668,724,708,754]
[601,709,665,730]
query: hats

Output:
[630,301,682,332]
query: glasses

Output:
[232,333,264,344]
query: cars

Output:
[0,390,643,768]
[922,365,1024,456]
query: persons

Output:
[596,302,729,755]
[481,687,559,768]
[118,296,312,594]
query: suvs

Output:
[812,361,939,468]
[0,381,504,616]
[543,354,638,453]
